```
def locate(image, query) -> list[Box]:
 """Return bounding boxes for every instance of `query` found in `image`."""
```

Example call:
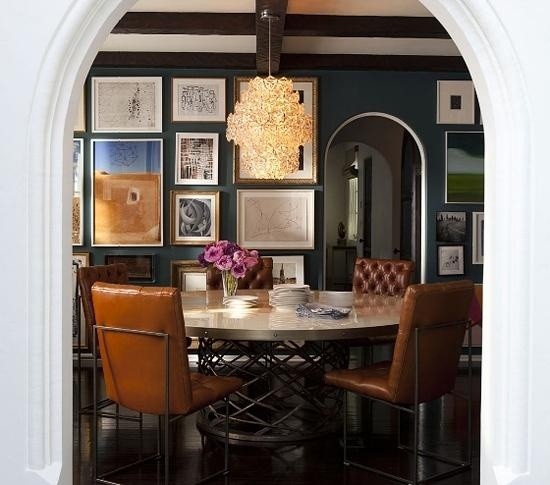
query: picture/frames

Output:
[469,210,484,266]
[261,255,304,350]
[237,190,315,249]
[432,76,478,127]
[101,252,156,287]
[443,128,485,205]
[170,190,220,246]
[232,74,319,185]
[72,85,86,133]
[175,132,219,185]
[91,77,162,133]
[435,244,467,277]
[72,253,90,347]
[71,138,84,246]
[90,138,164,248]
[170,76,226,124]
[169,261,249,350]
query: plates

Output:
[269,284,312,306]
[223,295,263,308]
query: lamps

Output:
[221,2,318,183]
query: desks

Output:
[330,244,358,278]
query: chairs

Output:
[87,277,248,485]
[70,257,192,454]
[342,255,414,365]
[318,276,476,485]
[195,255,278,395]
